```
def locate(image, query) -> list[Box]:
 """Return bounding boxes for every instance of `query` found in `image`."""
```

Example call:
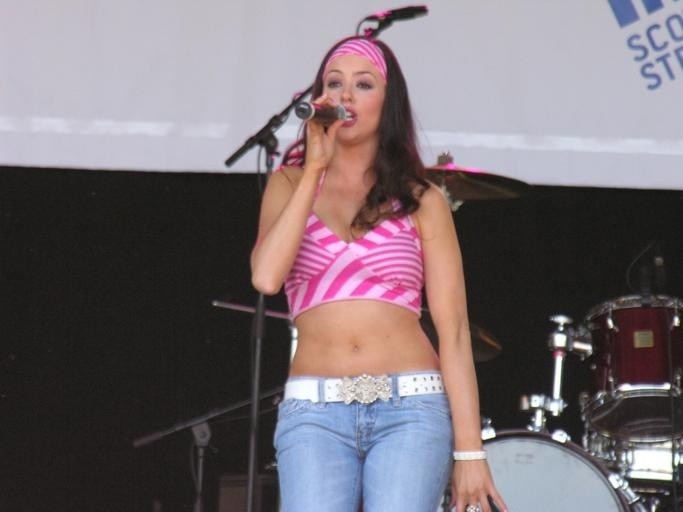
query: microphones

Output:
[297,99,347,123]
[364,3,429,20]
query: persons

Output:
[246,34,508,512]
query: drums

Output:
[440,428,629,512]
[579,292,683,431]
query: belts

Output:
[283,373,446,405]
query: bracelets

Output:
[452,449,487,462]
